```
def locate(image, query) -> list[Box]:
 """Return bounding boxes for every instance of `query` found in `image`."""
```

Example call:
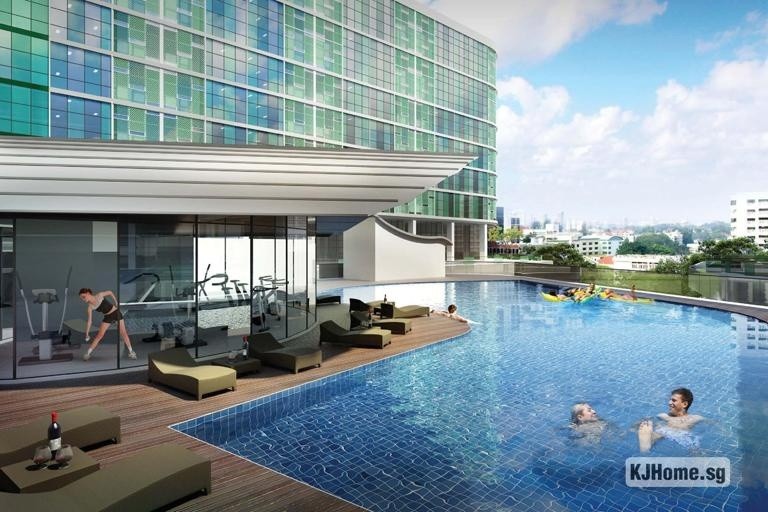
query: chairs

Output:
[147,295,430,401]
[0,403,211,511]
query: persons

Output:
[568,400,608,452]
[630,385,726,456]
[573,281,594,302]
[78,287,137,362]
[430,304,477,324]
[602,284,639,301]
[548,288,575,299]
